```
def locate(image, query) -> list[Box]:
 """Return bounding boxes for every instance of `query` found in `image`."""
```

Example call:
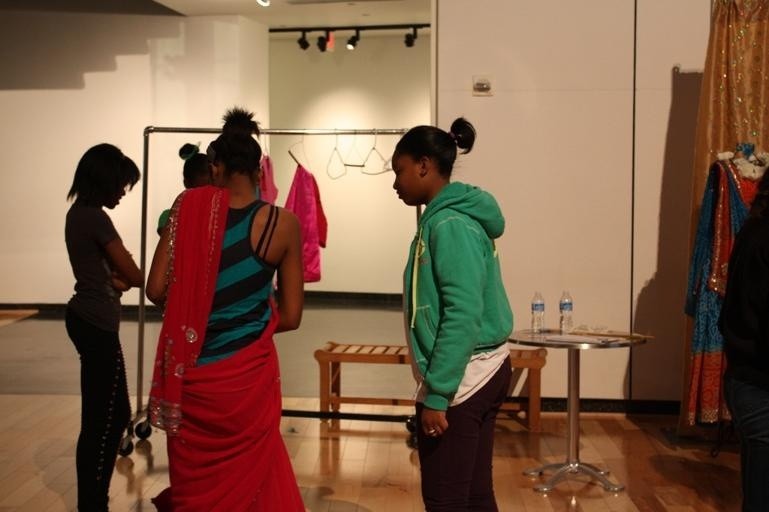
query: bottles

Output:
[530,290,546,339]
[559,288,576,339]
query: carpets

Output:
[0,309,422,398]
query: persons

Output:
[60,144,143,512]
[158,142,213,233]
[716,164,769,512]
[391,117,514,512]
[146,106,313,512]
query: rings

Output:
[428,429,435,434]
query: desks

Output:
[509,329,647,491]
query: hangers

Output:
[359,129,389,175]
[327,129,347,179]
[384,134,408,171]
[288,129,310,175]
[259,132,268,158]
[344,127,365,168]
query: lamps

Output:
[268,23,430,53]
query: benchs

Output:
[315,340,547,435]
